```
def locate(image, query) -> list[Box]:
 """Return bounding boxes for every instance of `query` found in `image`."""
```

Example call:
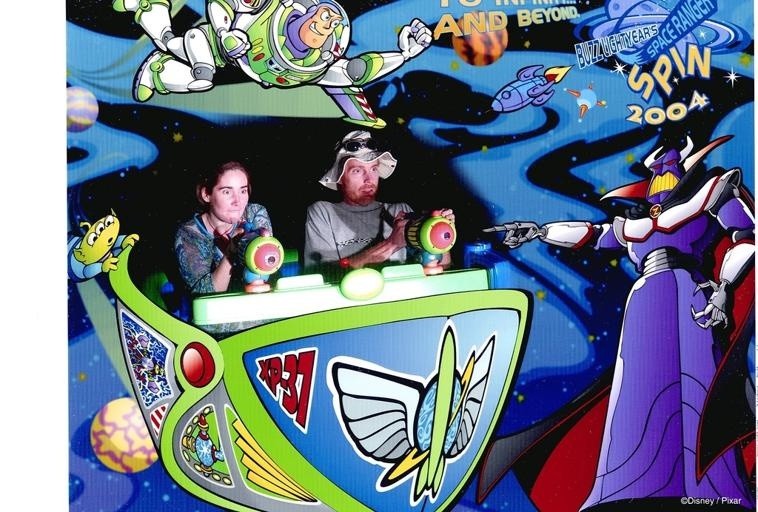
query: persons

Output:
[174,161,279,296]
[302,131,459,281]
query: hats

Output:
[317,129,398,191]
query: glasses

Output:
[343,137,377,152]
